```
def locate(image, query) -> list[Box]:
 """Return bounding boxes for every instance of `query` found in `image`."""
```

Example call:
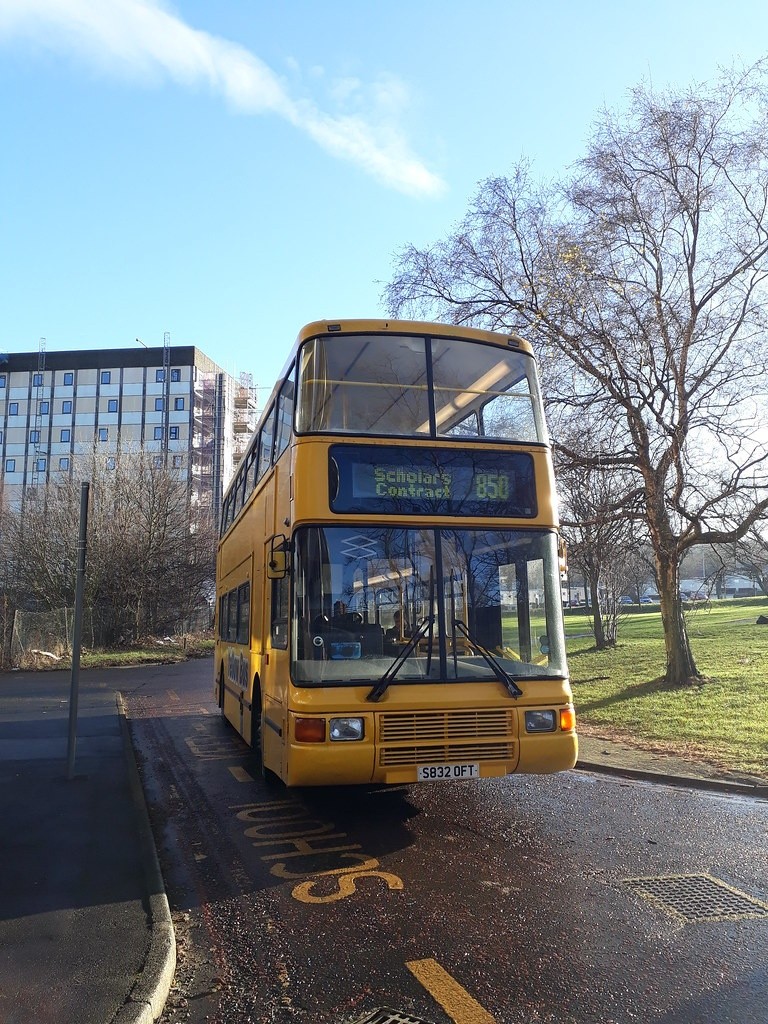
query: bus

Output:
[214,318,579,787]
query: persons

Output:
[387,610,425,639]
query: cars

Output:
[617,595,634,604]
[640,597,653,604]
[680,592,689,602]
[570,598,593,607]
